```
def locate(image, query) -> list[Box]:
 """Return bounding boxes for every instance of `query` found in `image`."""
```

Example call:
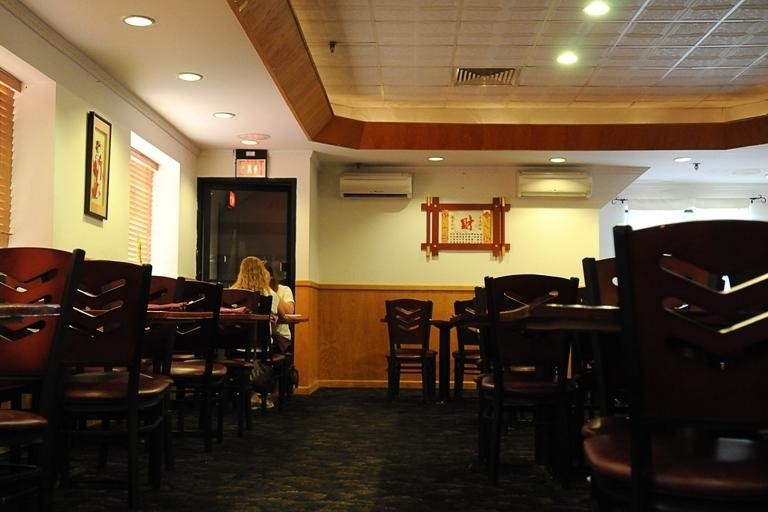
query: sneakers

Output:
[250,395,278,409]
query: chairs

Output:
[429,219,768,512]
[380,298,438,400]
[0,248,292,512]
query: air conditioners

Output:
[339,170,412,201]
[516,168,593,199]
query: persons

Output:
[227,255,286,412]
[263,258,296,404]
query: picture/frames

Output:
[84,110,112,220]
[421,195,512,257]
